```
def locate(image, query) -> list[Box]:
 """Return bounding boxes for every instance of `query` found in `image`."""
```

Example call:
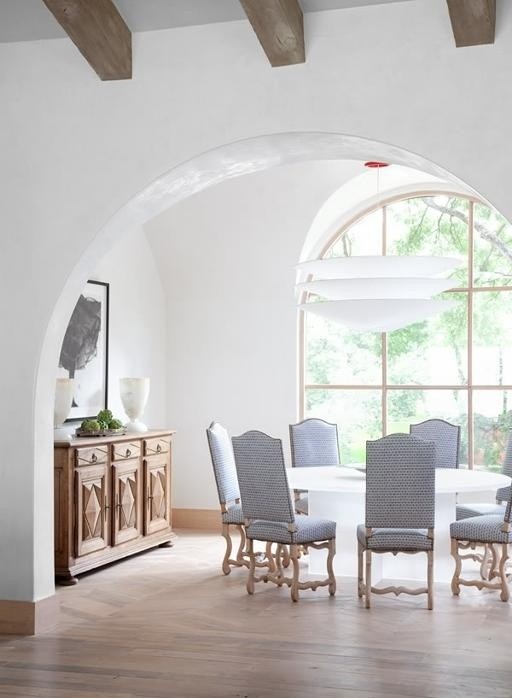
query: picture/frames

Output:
[56,279,111,419]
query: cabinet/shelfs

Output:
[54,428,178,587]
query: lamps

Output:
[295,160,465,334]
[118,377,151,435]
[54,377,74,441]
